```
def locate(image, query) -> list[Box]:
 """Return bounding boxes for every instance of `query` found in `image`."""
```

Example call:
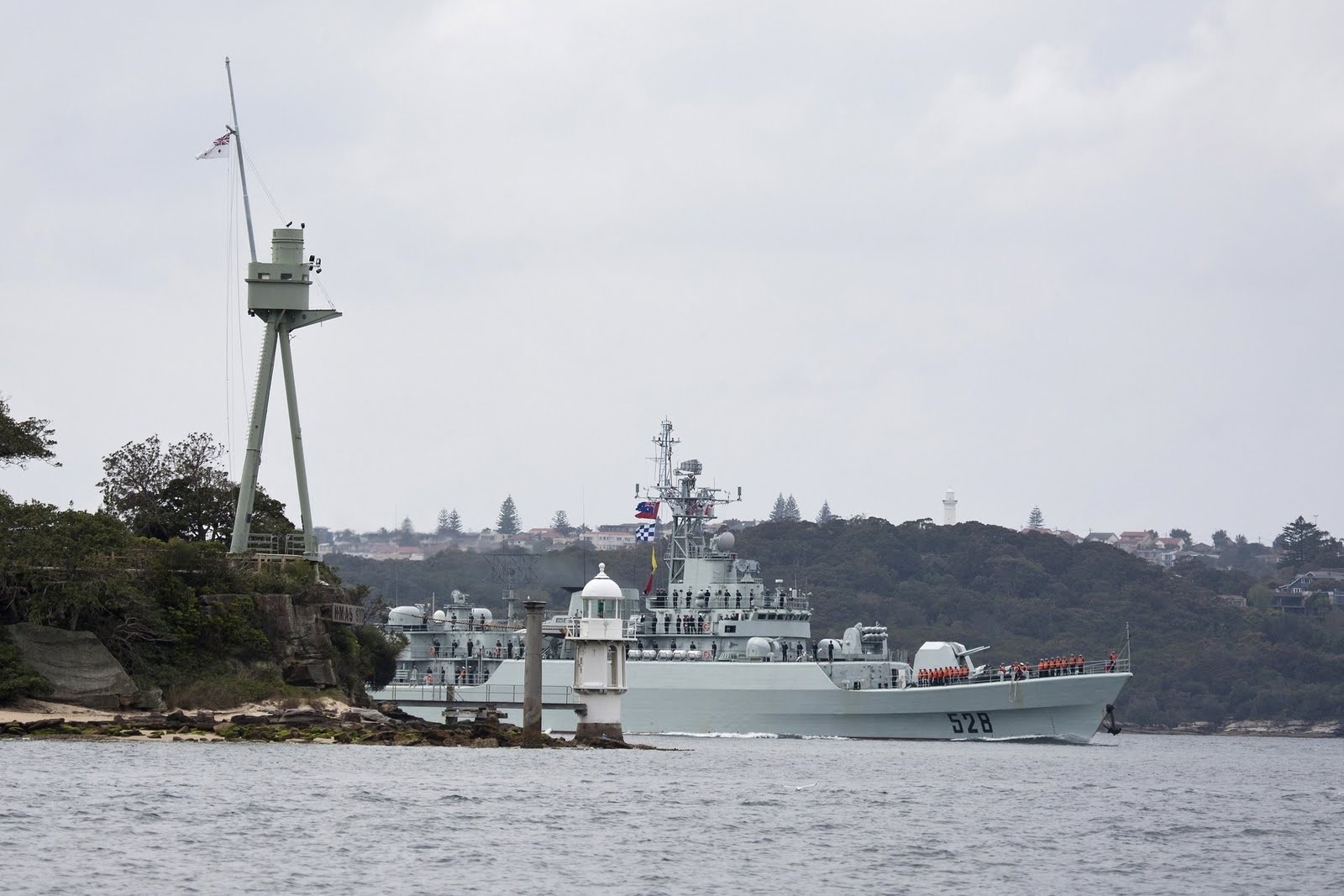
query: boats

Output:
[367,418,1135,747]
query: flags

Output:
[635,523,656,541]
[635,501,660,519]
[194,132,232,160]
[645,546,658,592]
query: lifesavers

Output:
[703,623,710,631]
[430,647,435,654]
[426,676,432,682]
[494,648,499,654]
[703,651,710,659]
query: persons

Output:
[704,589,710,608]
[918,664,969,688]
[828,641,834,661]
[812,640,818,662]
[782,641,788,662]
[750,589,755,609]
[1110,651,1116,673]
[797,640,803,662]
[672,589,678,609]
[736,589,742,609]
[685,589,692,608]
[1039,654,1085,678]
[1000,662,1032,681]
[656,588,667,607]
[399,612,737,685]
[779,590,786,609]
[724,589,731,609]
